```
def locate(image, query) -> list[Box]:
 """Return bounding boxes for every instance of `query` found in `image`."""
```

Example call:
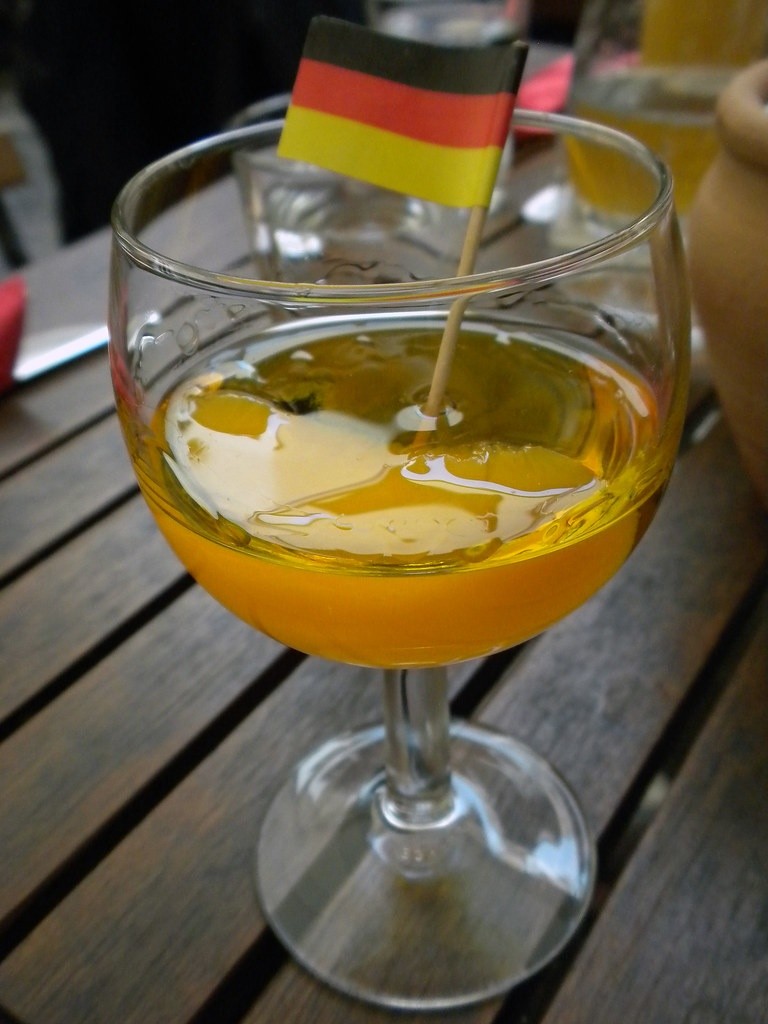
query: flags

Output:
[278,13,530,209]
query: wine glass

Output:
[108,106,688,1011]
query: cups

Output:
[228,93,512,324]
[558,68,727,339]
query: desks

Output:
[0,171,768,1024]
